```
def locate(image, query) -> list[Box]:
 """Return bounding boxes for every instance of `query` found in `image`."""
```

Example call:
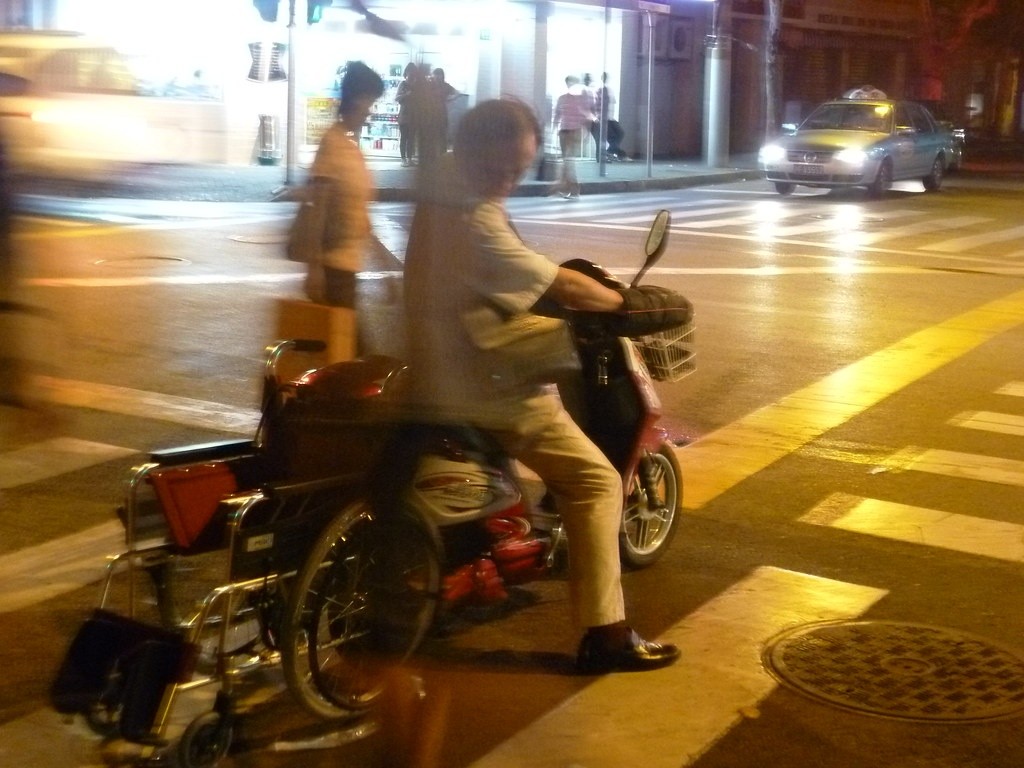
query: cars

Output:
[757,96,954,196]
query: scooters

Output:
[279,206,698,618]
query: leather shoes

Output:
[571,628,680,675]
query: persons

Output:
[395,63,459,167]
[302,63,384,309]
[551,73,632,200]
[404,98,681,672]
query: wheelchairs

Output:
[42,339,436,768]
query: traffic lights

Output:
[308,0,333,24]
[253,0,278,22]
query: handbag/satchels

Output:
[267,296,356,385]
